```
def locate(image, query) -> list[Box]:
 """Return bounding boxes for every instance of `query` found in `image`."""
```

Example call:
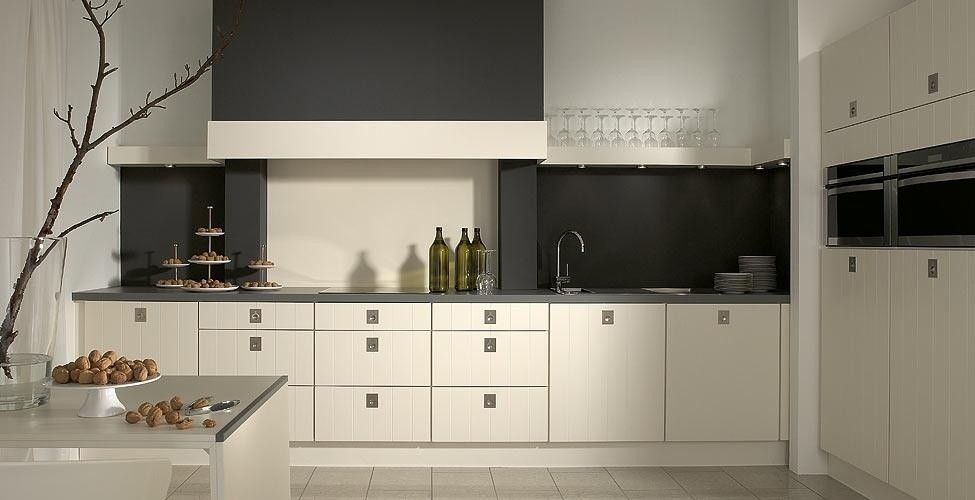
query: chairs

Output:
[0,460,173,500]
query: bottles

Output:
[469,228,490,292]
[453,227,474,292]
[428,226,450,293]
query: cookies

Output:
[156,227,281,289]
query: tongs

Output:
[178,394,242,418]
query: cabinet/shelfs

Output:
[84,302,199,378]
[819,247,975,499]
[818,1,974,170]
[431,302,549,469]
[315,302,431,468]
[198,302,315,467]
[549,303,780,468]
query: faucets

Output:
[556,230,585,275]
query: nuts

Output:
[51,349,218,431]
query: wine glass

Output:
[475,249,498,297]
[546,102,726,152]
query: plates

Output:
[738,254,779,296]
[713,270,754,297]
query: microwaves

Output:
[819,152,891,247]
[891,137,975,248]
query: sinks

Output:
[552,287,596,292]
